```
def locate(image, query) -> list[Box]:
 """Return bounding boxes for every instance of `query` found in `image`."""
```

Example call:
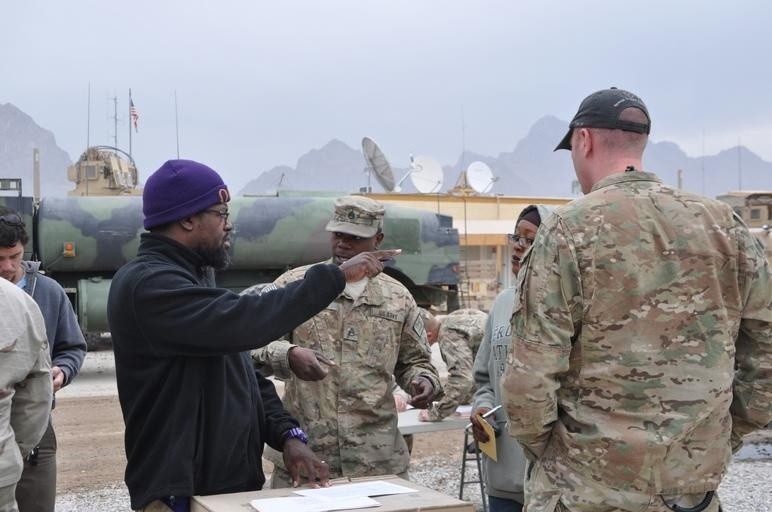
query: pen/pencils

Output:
[464,404,502,429]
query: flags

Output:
[131,99,141,134]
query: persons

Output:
[468,202,560,511]
[415,305,489,422]
[106,159,406,510]
[237,194,445,490]
[0,276,58,511]
[1,207,89,511]
[499,85,770,511]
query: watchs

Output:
[279,426,309,446]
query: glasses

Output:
[507,234,534,248]
[206,208,229,224]
[0,214,23,226]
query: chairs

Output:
[457,419,489,512]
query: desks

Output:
[385,402,471,460]
[192,474,477,512]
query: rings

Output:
[320,460,326,463]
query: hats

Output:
[325,198,385,239]
[553,87,651,152]
[143,161,230,230]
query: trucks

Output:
[714,189,772,269]
[66,79,145,196]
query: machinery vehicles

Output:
[0,176,462,352]
